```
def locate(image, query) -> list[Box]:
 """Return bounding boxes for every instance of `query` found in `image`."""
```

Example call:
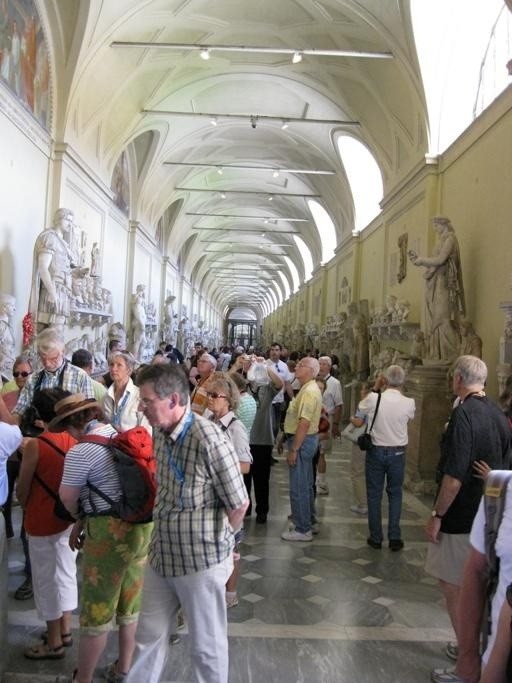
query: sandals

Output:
[38,633,76,651]
[23,642,69,665]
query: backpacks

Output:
[77,424,157,523]
[35,434,77,523]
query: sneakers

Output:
[279,517,322,531]
[255,513,271,530]
[388,537,405,551]
[14,578,37,603]
[368,536,386,552]
[280,527,314,540]
[430,665,462,682]
[444,641,468,662]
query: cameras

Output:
[242,354,257,361]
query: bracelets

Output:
[333,421,339,425]
[370,387,378,393]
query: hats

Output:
[47,393,101,433]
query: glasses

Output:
[136,395,165,411]
[38,355,62,364]
[13,371,32,379]
[206,391,230,401]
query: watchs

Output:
[431,510,443,520]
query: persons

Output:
[497,363,511,411]
[0,376,24,679]
[482,581,512,682]
[393,333,428,365]
[3,354,39,414]
[15,388,78,659]
[72,348,108,405]
[316,356,344,495]
[267,343,294,436]
[234,373,257,436]
[281,357,322,541]
[131,284,147,360]
[182,313,225,353]
[162,296,176,344]
[108,340,123,353]
[357,365,416,550]
[408,215,466,365]
[425,355,512,682]
[226,354,283,525]
[472,458,492,482]
[92,336,107,371]
[259,301,367,362]
[444,391,464,432]
[202,375,253,609]
[48,392,154,683]
[66,333,89,351]
[371,298,410,321]
[341,378,380,515]
[124,362,250,683]
[461,320,482,356]
[189,352,218,416]
[33,208,75,329]
[155,337,320,355]
[73,243,117,311]
[105,350,152,439]
[11,327,96,602]
[276,377,331,518]
[1,294,17,383]
[457,470,511,682]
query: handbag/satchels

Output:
[358,391,382,451]
